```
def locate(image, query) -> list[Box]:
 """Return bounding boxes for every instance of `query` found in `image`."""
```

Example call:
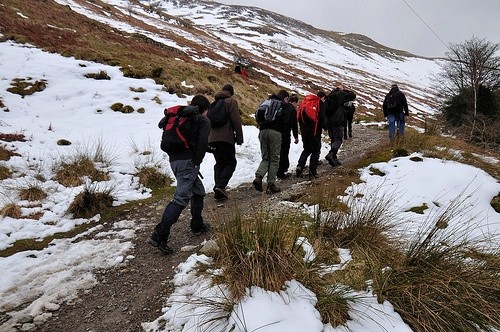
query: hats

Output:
[223,84,234,95]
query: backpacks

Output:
[299,94,320,121]
[256,99,284,124]
[387,90,400,108]
[327,89,342,110]
[159,104,200,151]
[207,99,230,128]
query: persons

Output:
[146,95,211,255]
[253,84,356,195]
[206,85,243,201]
[234,65,246,76]
[382,84,409,144]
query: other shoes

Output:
[253,178,263,192]
[276,173,288,179]
[325,154,336,167]
[266,183,281,195]
[332,160,342,167]
[309,168,317,177]
[212,186,228,201]
[296,166,304,178]
[348,132,352,138]
[344,134,347,140]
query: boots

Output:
[189,215,210,236]
[147,223,173,253]
